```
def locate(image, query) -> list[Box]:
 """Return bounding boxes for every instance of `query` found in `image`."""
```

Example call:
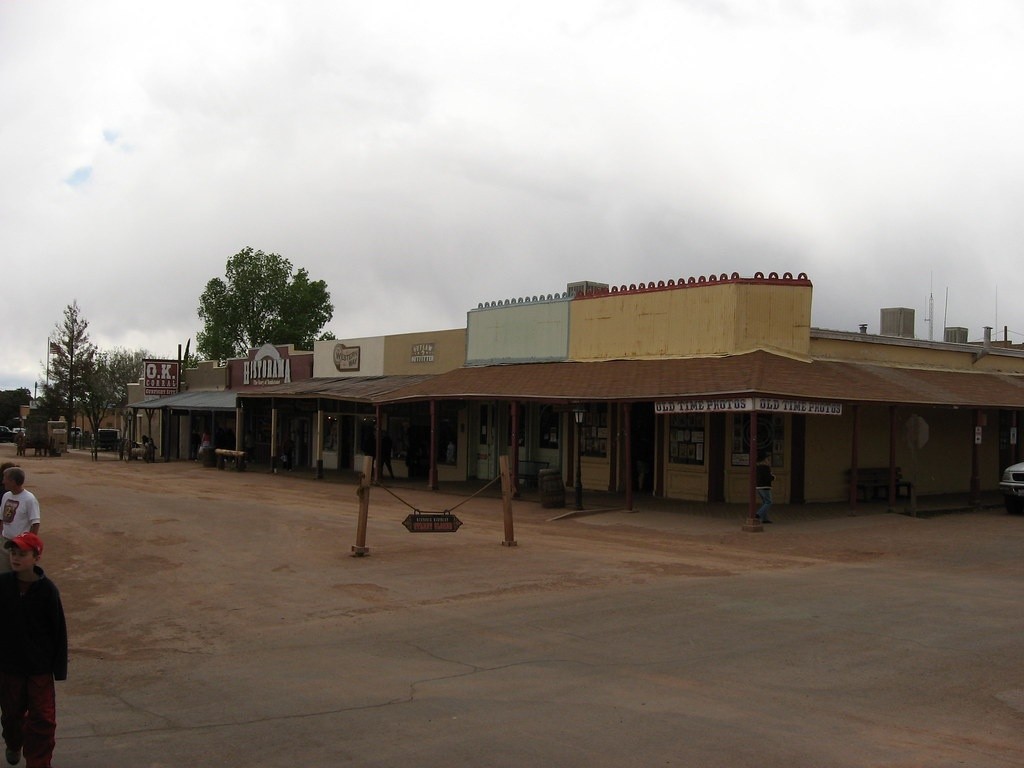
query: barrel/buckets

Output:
[539,469,566,509]
[203,448,217,467]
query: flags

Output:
[50,343,66,354]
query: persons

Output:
[0,467,40,541]
[399,445,416,480]
[0,463,20,506]
[755,452,775,523]
[245,430,256,463]
[191,428,210,458]
[0,531,68,768]
[364,432,376,476]
[381,431,394,479]
[283,435,295,471]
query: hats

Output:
[4,532,43,555]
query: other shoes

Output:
[755,514,760,519]
[762,520,772,524]
[6,747,21,765]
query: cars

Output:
[998,463,1024,516]
[71,427,82,438]
[0,426,18,442]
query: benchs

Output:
[518,459,551,488]
[844,466,913,501]
